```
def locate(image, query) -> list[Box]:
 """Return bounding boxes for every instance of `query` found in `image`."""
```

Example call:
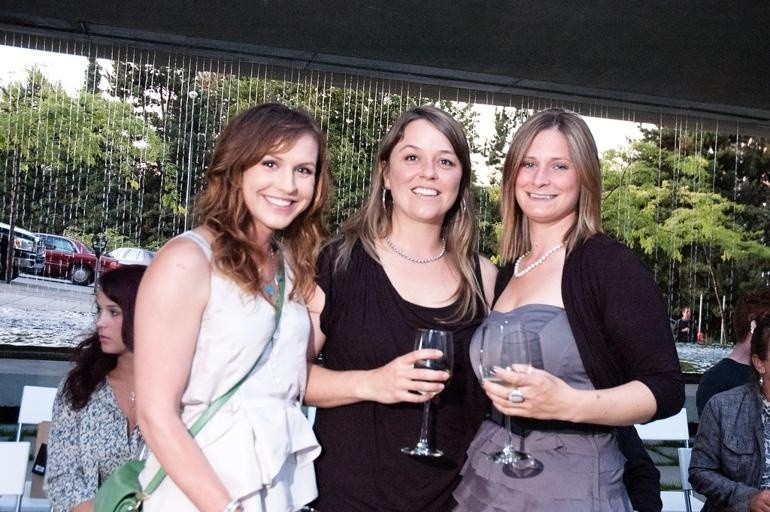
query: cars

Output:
[92,246,156,277]
[0,222,46,281]
[30,232,119,286]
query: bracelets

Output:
[223,495,245,511]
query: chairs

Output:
[634,407,692,512]
[677,446,704,512]
[16,386,62,445]
[1,443,32,512]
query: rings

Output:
[507,384,525,404]
[527,362,533,375]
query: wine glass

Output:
[479,317,523,467]
[495,329,547,480]
[398,326,454,458]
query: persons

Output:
[40,263,150,511]
[449,107,687,512]
[694,287,770,420]
[298,105,500,511]
[673,305,698,343]
[688,317,770,512]
[132,103,337,511]
[0,233,16,281]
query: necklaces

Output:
[513,240,565,279]
[110,371,135,403]
[381,227,447,264]
[241,246,277,295]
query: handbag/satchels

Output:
[91,459,145,512]
